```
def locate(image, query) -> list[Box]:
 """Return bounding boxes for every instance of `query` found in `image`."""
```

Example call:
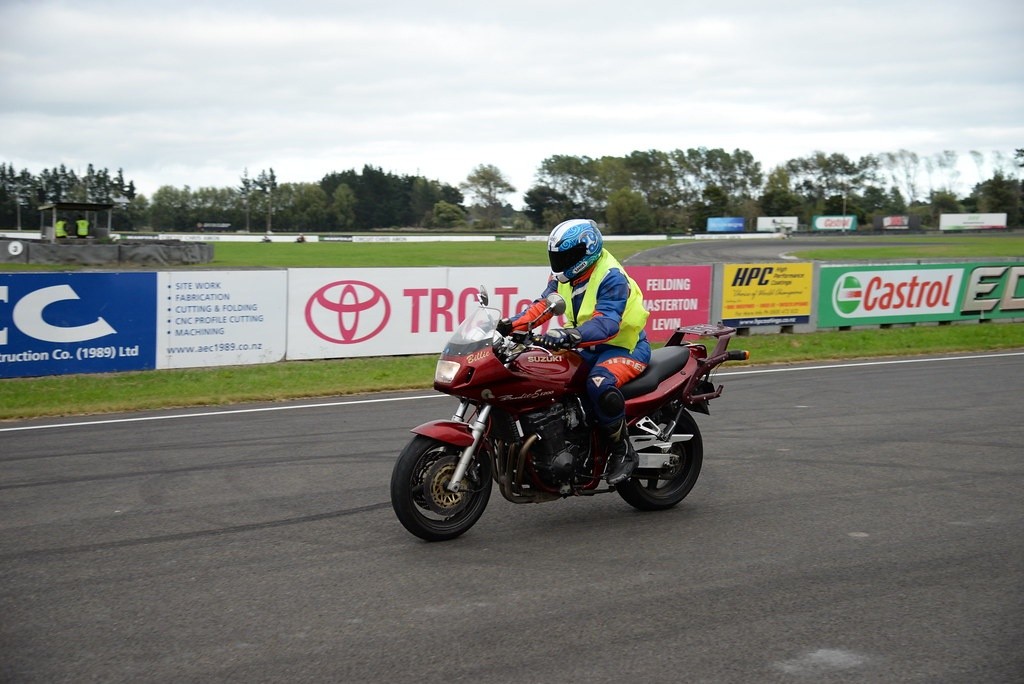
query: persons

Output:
[55,217,70,239]
[504,218,651,493]
[75,215,91,238]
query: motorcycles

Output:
[390,285,751,541]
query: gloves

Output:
[540,328,581,350]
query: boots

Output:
[604,418,639,484]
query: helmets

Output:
[548,219,602,284]
